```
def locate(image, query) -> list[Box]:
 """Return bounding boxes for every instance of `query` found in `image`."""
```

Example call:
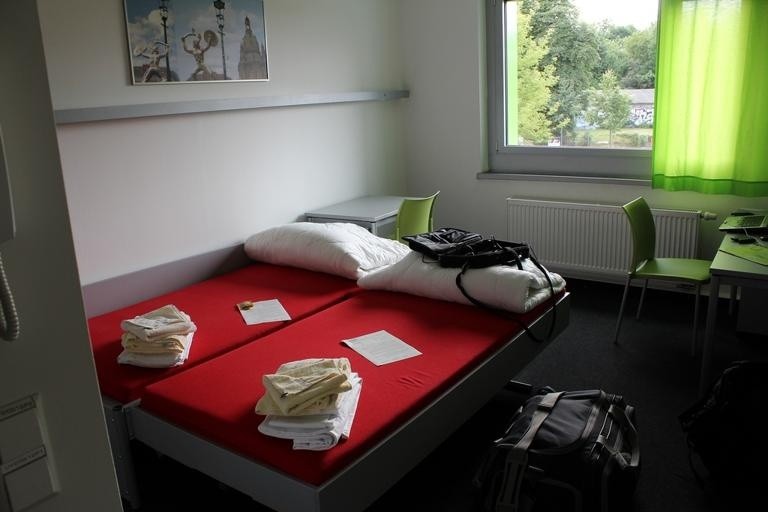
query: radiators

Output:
[505,197,717,287]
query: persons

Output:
[138,46,172,83]
[182,35,214,80]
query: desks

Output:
[304,195,404,234]
[698,231,768,400]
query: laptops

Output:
[718,214,768,232]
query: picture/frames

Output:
[122,0,269,87]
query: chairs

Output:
[612,197,714,358]
[391,190,440,240]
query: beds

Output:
[129,292,569,512]
[88,263,373,508]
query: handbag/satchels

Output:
[401,227,530,269]
[471,385,641,512]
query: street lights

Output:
[213,0,227,80]
[158,2,171,82]
